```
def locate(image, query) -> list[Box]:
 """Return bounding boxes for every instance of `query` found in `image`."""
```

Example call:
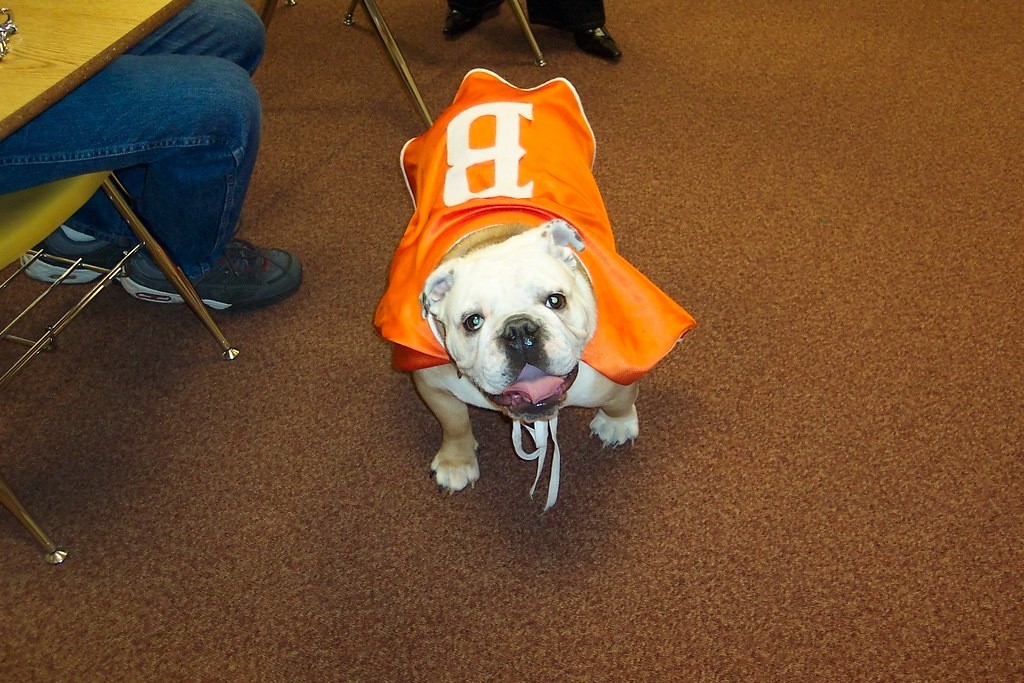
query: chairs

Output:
[0,171,239,562]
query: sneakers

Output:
[20,224,125,284]
[119,217,303,312]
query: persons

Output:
[1,0,301,314]
[444,0,623,64]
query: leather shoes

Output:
[442,7,499,37]
[574,25,623,61]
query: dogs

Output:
[389,67,641,500]
